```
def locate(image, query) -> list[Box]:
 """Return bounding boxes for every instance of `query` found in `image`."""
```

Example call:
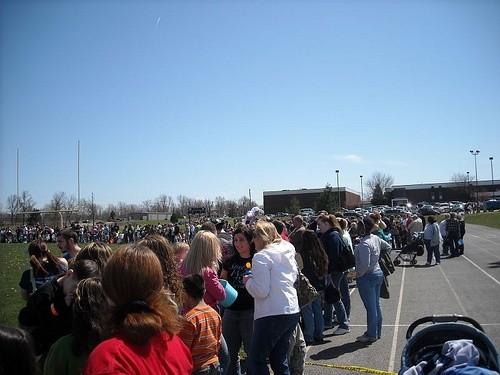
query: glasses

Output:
[252,233,260,239]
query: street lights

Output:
[470,149,480,203]
[489,156,494,199]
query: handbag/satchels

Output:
[417,245,424,256]
[324,280,341,305]
[380,278,390,299]
[109,239,114,244]
[327,230,356,274]
[383,252,395,272]
[294,272,321,309]
[457,238,463,249]
[378,256,391,278]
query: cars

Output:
[341,201,464,216]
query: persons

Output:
[0,208,439,375]
[440,200,481,258]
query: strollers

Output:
[393,236,423,266]
[399,314,500,375]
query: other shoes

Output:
[357,334,381,342]
[450,254,459,257]
[333,327,351,335]
[334,314,350,322]
[426,262,430,266]
[441,253,449,256]
[435,262,439,265]
[324,324,334,330]
[305,338,324,345]
[458,252,464,255]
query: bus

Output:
[299,208,314,216]
